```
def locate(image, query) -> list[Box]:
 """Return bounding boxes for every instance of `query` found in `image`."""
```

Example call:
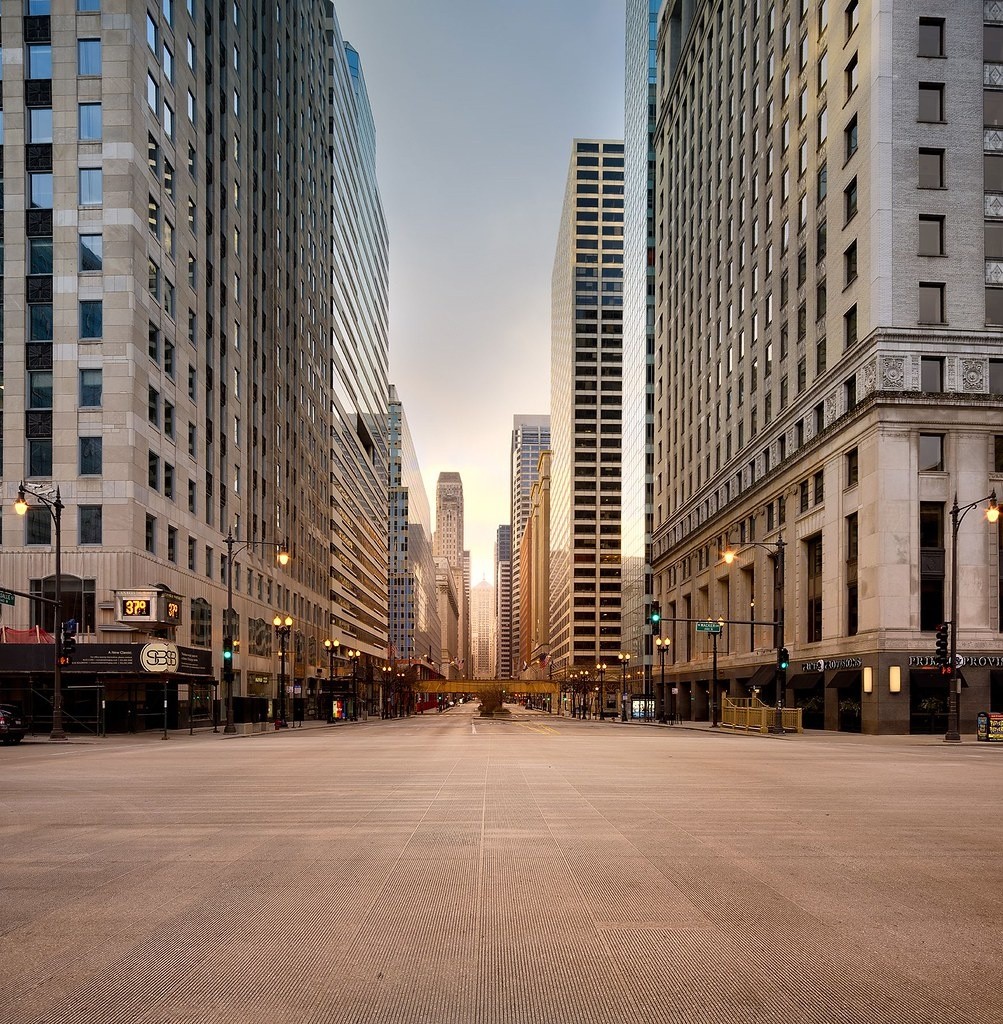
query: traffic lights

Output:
[223,638,232,672]
[59,654,70,666]
[64,621,76,655]
[935,622,947,666]
[651,600,660,633]
[779,647,788,671]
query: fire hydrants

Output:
[275,717,281,730]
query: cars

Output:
[0,703,26,743]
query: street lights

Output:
[569,671,588,719]
[619,653,631,721]
[223,525,289,735]
[725,529,790,734]
[14,480,69,741]
[347,649,360,721]
[946,487,1000,740]
[597,663,607,720]
[383,666,392,718]
[273,614,292,727]
[706,615,724,728]
[655,636,670,724]
[325,638,339,724]
